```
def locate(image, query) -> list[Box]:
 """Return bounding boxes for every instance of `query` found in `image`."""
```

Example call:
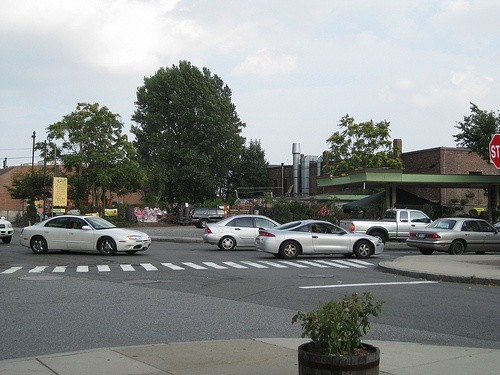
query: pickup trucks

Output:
[339,207,434,244]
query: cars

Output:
[19,214,152,256]
[203,213,281,251]
[406,217,500,255]
[255,220,385,260]
[0,216,15,244]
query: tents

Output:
[343,188,431,213]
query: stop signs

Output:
[489,134,500,169]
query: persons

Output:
[72,222,77,229]
[312,226,317,232]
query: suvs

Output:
[189,205,225,229]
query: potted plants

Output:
[450,197,459,203]
[291,291,387,375]
[465,191,475,198]
